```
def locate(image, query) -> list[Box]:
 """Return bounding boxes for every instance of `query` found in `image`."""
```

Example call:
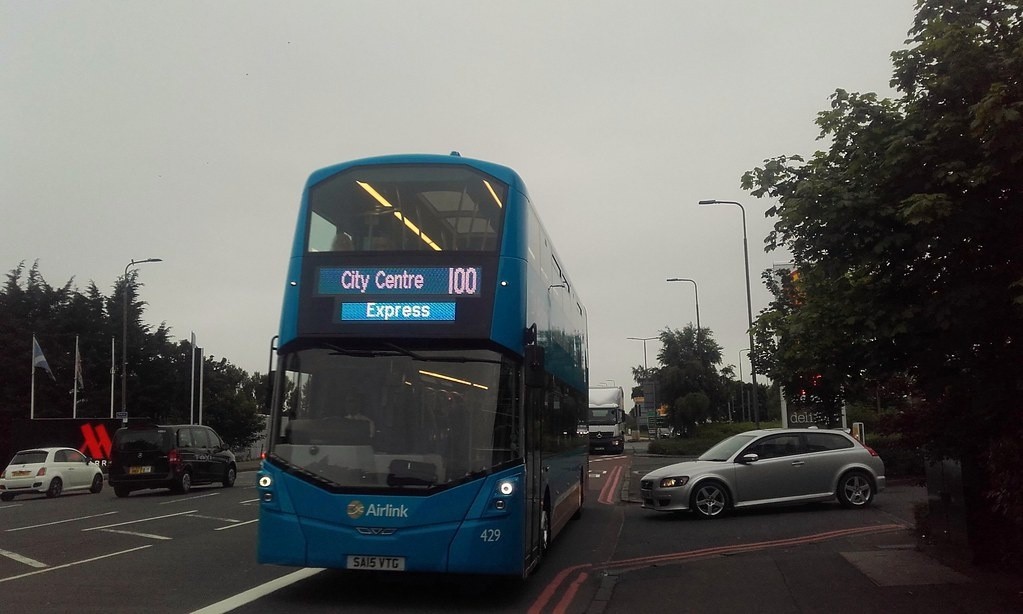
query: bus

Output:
[256,151,589,582]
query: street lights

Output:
[739,348,751,423]
[665,278,703,382]
[698,199,760,430]
[626,337,659,372]
[119,257,163,429]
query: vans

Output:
[108,424,238,498]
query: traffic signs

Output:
[116,411,128,419]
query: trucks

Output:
[588,386,625,455]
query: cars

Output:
[639,429,885,521]
[0,446,105,501]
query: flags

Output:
[75,337,83,386]
[32,334,56,381]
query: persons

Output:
[344,390,376,436]
[330,233,354,251]
